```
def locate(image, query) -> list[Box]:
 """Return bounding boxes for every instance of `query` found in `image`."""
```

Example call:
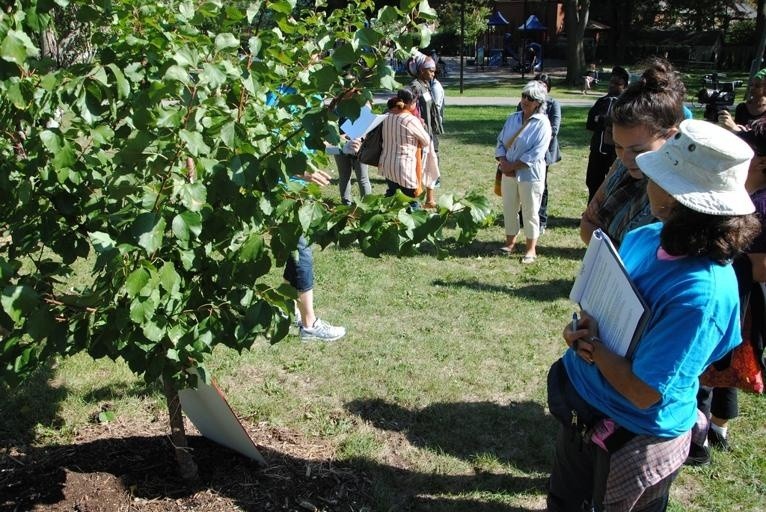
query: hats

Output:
[634,118,757,216]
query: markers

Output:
[573,313,577,359]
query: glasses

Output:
[522,93,539,102]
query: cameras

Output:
[695,73,744,124]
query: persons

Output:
[516,73,562,235]
[243,45,348,341]
[405,50,445,209]
[377,88,431,214]
[545,117,756,512]
[582,64,599,94]
[683,110,766,468]
[494,81,552,265]
[716,66,766,158]
[323,66,373,206]
[578,57,687,252]
[582,66,630,218]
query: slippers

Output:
[520,256,536,263]
[491,246,514,256]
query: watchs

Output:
[583,336,603,356]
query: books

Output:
[568,229,650,365]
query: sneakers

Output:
[706,424,730,451]
[298,317,346,342]
[684,443,711,466]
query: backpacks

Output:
[494,115,531,197]
